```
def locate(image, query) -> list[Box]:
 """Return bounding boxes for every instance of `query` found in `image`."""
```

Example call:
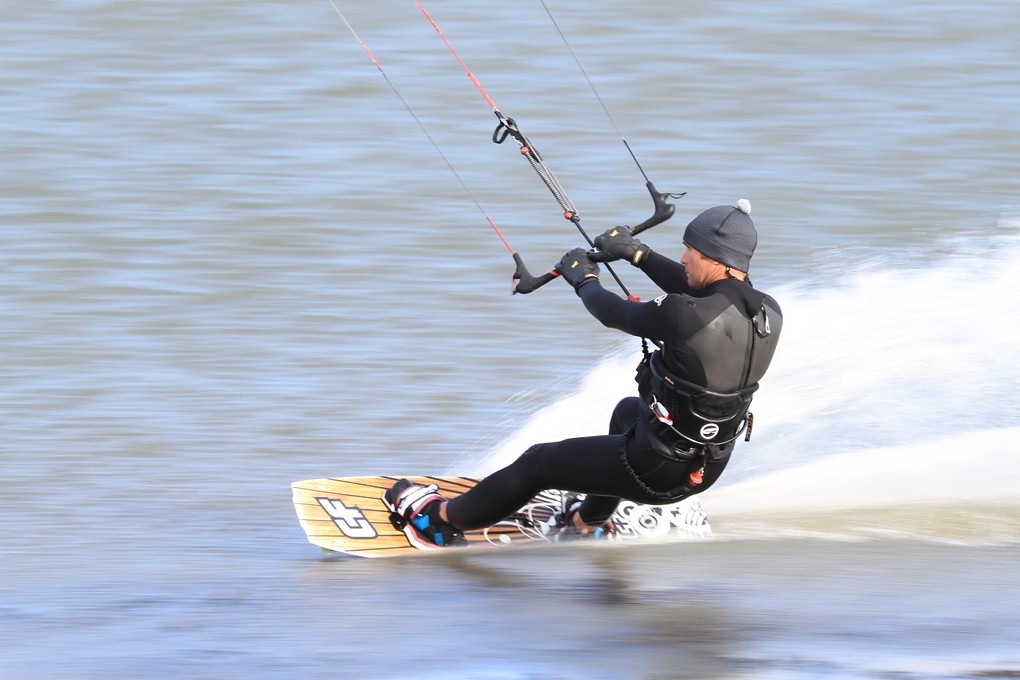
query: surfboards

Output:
[290,476,712,559]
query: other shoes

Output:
[393,479,458,544]
[563,491,591,539]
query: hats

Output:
[683,199,757,274]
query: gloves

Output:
[590,226,649,267]
[555,248,600,296]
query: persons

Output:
[394,199,783,544]
